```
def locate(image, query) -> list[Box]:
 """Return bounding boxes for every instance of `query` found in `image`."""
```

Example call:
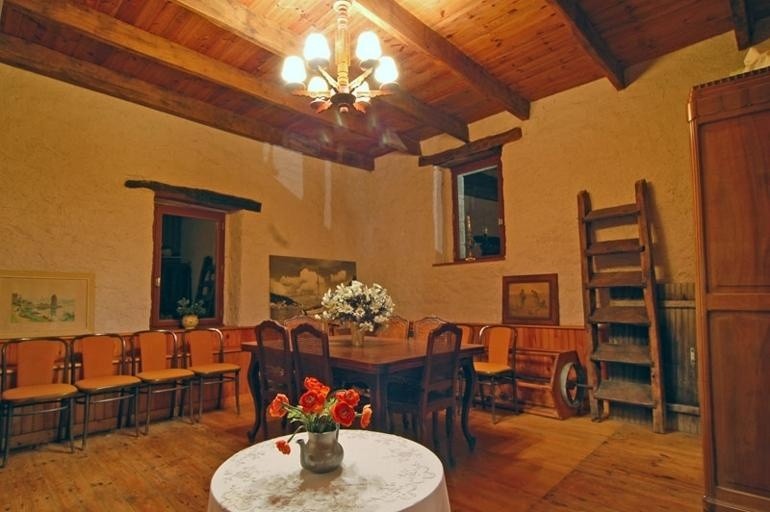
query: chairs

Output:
[182,327,241,422]
[70,332,141,451]
[290,323,354,429]
[129,329,194,436]
[376,314,410,338]
[449,321,475,416]
[387,321,463,472]
[283,315,328,339]
[0,334,78,468]
[412,316,453,341]
[466,324,518,424]
[253,318,298,441]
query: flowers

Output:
[314,279,396,333]
[266,375,374,454]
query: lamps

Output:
[281,0,401,117]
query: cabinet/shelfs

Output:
[685,66,770,511]
[160,260,190,320]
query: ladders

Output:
[578,179,669,434]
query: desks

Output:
[205,429,452,511]
[240,334,485,452]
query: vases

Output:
[349,325,366,347]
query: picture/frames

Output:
[502,272,561,325]
[0,268,96,343]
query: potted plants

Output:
[173,297,207,330]
